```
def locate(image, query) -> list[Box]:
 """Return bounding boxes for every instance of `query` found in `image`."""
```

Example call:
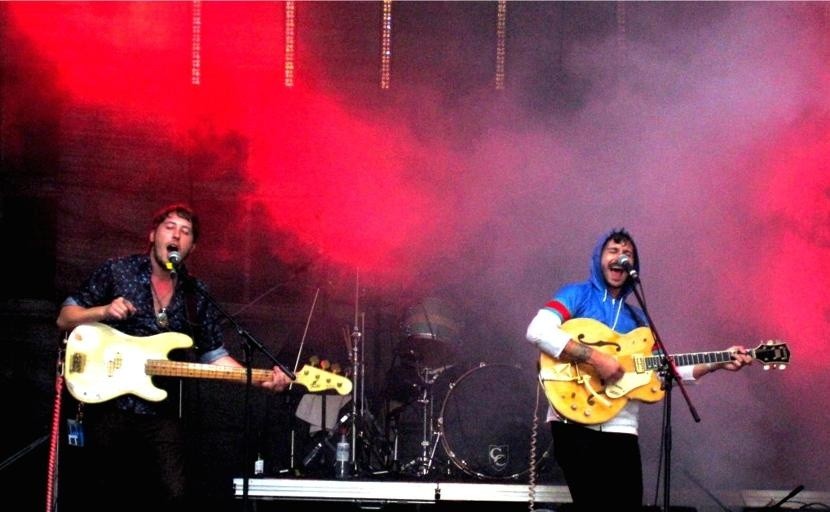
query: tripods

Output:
[398,365,445,475]
[296,267,403,474]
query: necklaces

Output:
[151,276,172,330]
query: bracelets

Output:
[707,363,716,371]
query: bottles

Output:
[335,429,352,480]
[253,451,265,478]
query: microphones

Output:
[617,254,641,284]
[168,251,182,279]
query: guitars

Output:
[540,318,790,425]
[64,321,353,403]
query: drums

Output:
[438,361,554,480]
[399,298,459,352]
[389,365,425,396]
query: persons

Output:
[55,204,294,512]
[523,225,754,512]
[295,394,356,479]
